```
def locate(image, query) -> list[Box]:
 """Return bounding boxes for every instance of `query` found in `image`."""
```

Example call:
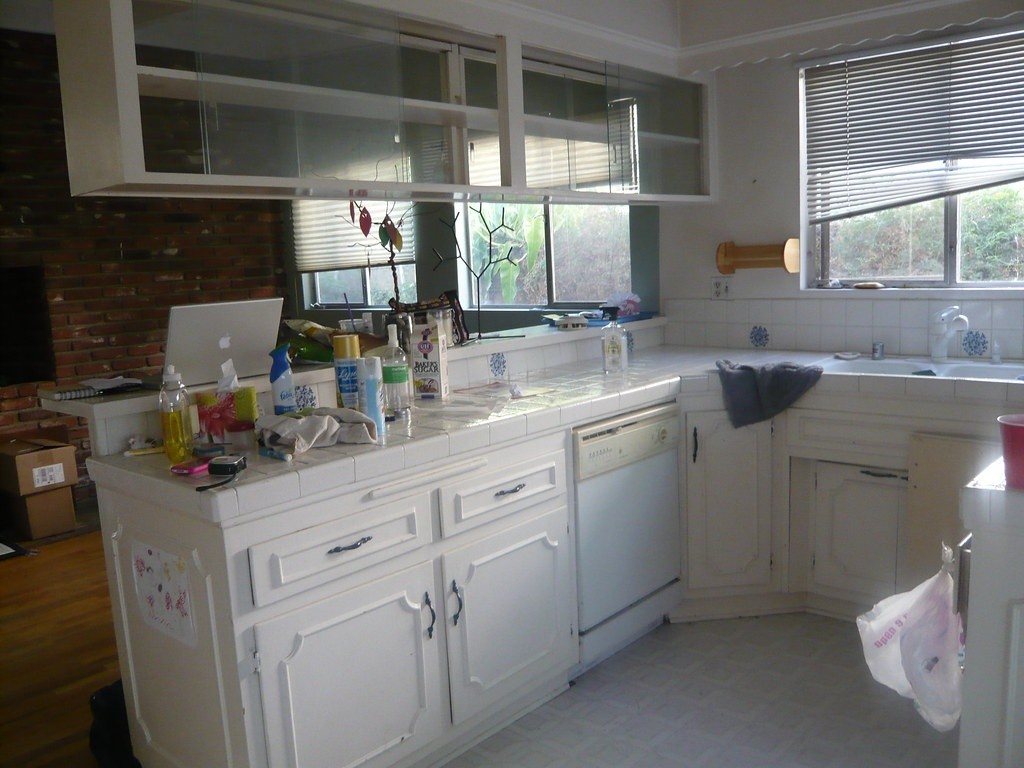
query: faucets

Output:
[930,305,968,362]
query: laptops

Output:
[122,297,286,391]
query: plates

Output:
[836,351,861,359]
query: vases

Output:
[386,311,411,352]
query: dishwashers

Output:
[572,401,681,633]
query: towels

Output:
[254,406,379,457]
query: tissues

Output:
[197,358,257,421]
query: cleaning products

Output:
[599,307,633,372]
[159,365,192,465]
[381,323,410,411]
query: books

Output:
[36,384,100,400]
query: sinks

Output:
[822,360,921,376]
[944,365,1023,379]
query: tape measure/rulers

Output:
[207,453,247,475]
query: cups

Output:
[997,414,1024,489]
[362,312,374,332]
[872,342,884,359]
[427,309,454,347]
[230,420,256,449]
[339,319,363,330]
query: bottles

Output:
[159,365,194,462]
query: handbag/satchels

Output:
[856,543,964,732]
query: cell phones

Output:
[171,455,213,474]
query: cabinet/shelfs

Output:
[952,529,1023,768]
[787,407,912,623]
[96,427,576,768]
[677,391,787,623]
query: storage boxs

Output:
[0,436,80,540]
[411,310,449,398]
